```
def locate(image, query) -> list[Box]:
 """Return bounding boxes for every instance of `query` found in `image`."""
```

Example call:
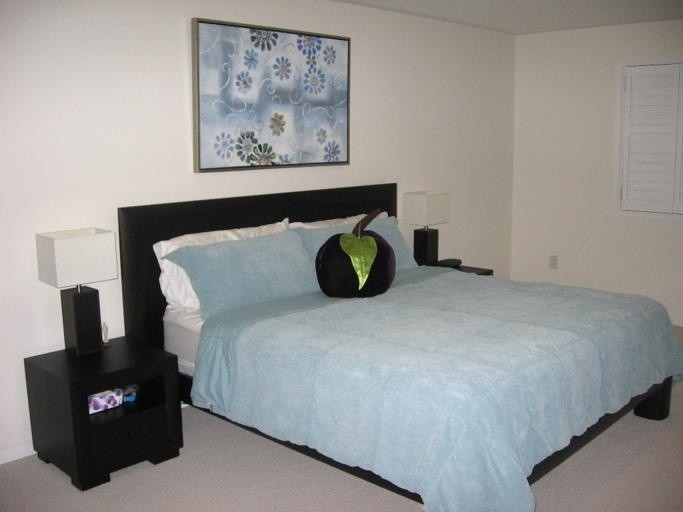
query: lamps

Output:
[403,189,453,266]
[33,222,123,359]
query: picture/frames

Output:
[190,16,352,176]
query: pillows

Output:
[152,210,419,311]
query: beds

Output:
[116,181,681,512]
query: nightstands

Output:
[460,264,496,276]
[21,332,187,493]
